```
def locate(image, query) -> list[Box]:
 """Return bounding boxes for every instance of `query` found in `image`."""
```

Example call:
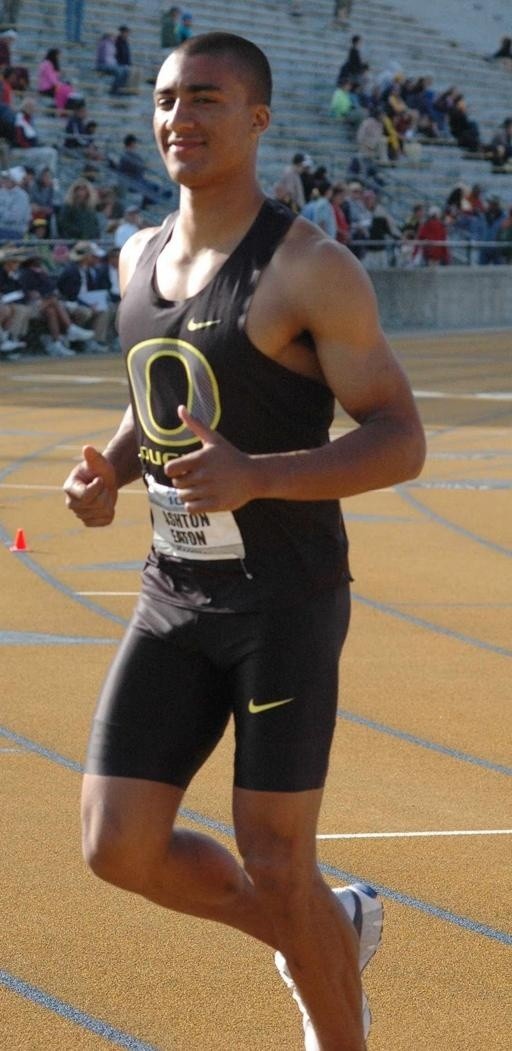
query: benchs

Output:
[0,2,512,259]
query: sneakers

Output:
[274,884,385,1050]
[0,324,111,361]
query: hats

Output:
[0,246,28,262]
[69,241,95,260]
[90,241,105,258]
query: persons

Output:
[63,32,427,1051]
